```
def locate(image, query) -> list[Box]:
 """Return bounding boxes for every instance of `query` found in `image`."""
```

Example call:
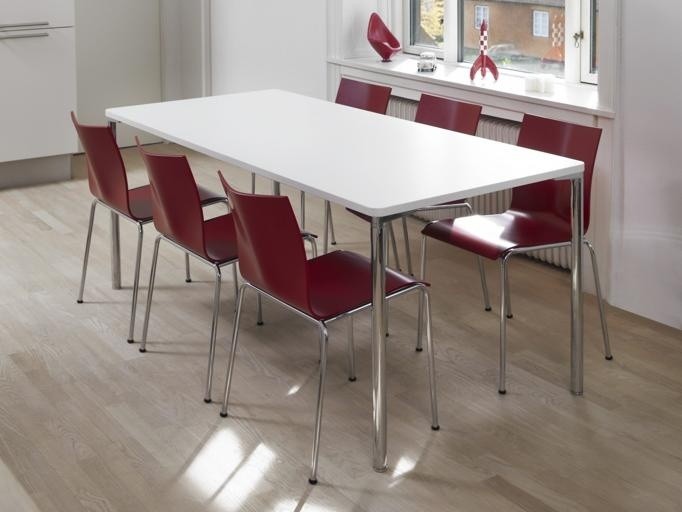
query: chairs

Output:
[251,76,392,242]
[411,112,615,394]
[206,167,443,484]
[323,91,483,281]
[132,135,264,404]
[67,108,191,345]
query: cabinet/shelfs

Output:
[0,0,78,171]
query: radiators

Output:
[334,77,598,270]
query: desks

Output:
[105,89,588,475]
